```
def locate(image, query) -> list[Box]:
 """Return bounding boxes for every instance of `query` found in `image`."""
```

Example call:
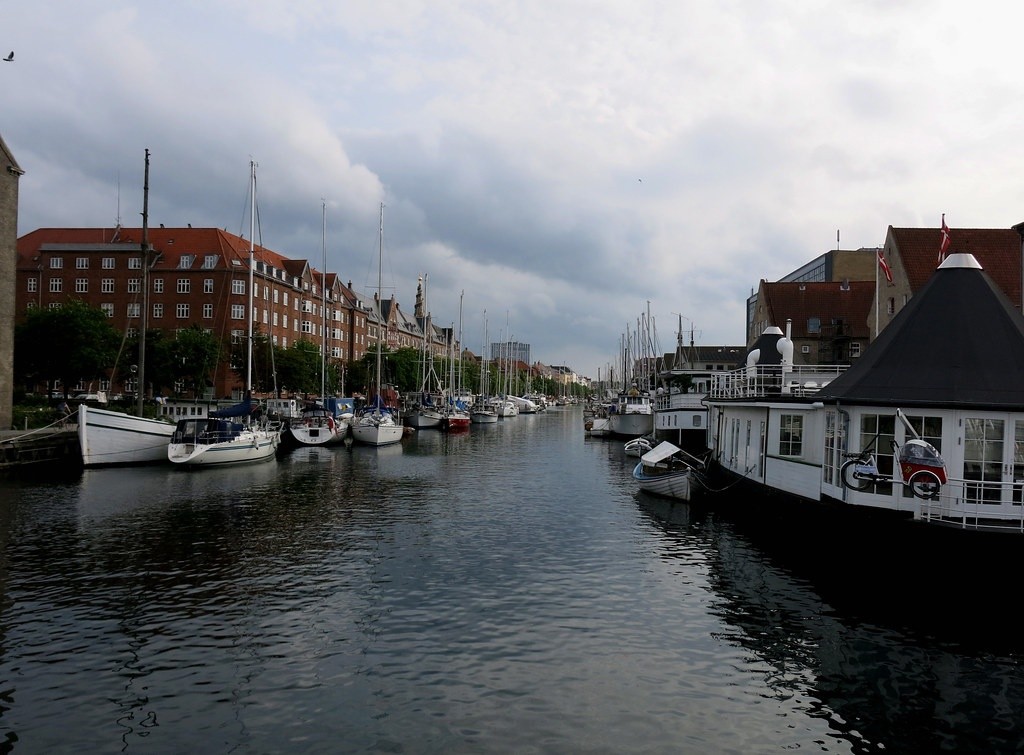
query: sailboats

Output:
[165,157,285,467]
[286,198,349,446]
[350,201,406,447]
[70,148,199,468]
[405,274,660,442]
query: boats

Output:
[625,437,650,448]
[624,441,652,458]
[634,488,707,535]
[631,440,710,503]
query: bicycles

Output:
[840,438,949,499]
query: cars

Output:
[45,391,64,400]
